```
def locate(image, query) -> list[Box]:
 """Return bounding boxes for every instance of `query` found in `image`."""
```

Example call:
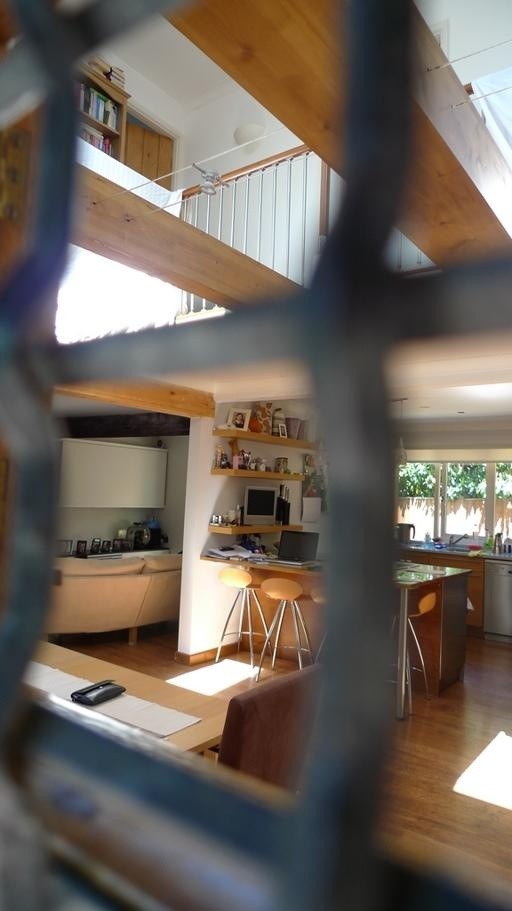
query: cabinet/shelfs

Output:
[77,61,131,164]
[399,552,485,629]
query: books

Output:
[73,52,127,157]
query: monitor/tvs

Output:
[243,486,278,525]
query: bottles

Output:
[472,524,479,541]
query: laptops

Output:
[261,530,320,565]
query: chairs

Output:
[199,663,320,793]
[389,592,436,715]
[215,568,327,683]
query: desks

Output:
[21,641,229,753]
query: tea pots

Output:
[394,523,415,544]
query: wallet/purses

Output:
[71,678,125,705]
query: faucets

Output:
[450,533,469,545]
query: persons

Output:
[231,413,246,429]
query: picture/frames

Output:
[57,538,134,556]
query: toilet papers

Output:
[302,497,322,523]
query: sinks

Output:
[446,547,470,553]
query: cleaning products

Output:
[484,528,490,547]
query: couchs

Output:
[41,554,182,647]
